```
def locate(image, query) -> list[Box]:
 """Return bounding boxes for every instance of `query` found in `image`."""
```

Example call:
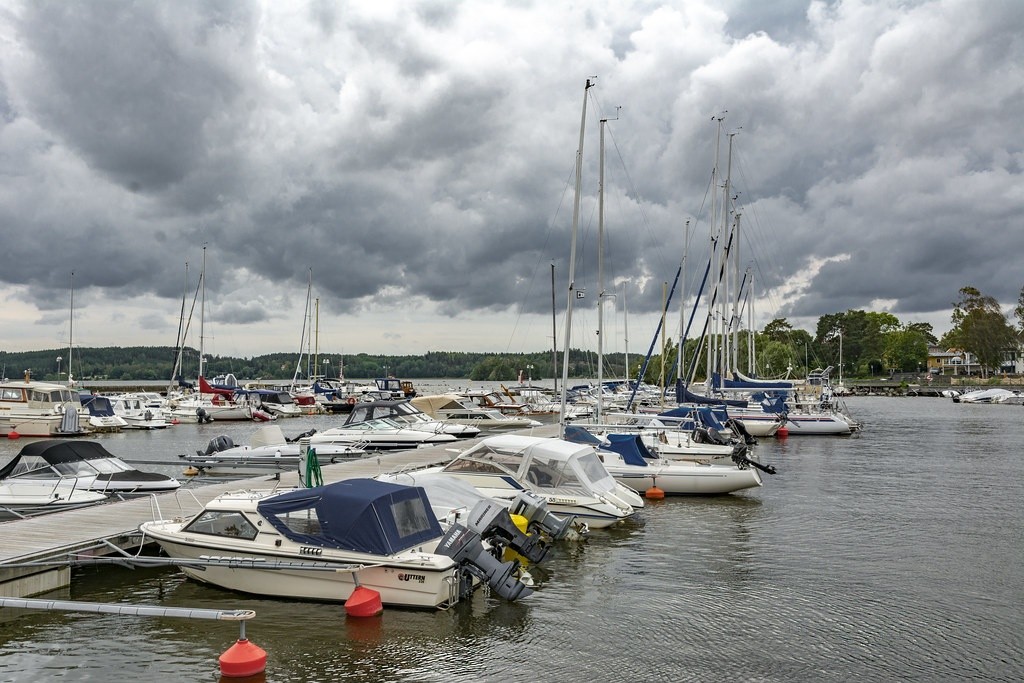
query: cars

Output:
[929,367,939,375]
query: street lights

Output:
[323,358,330,378]
[527,364,533,389]
[638,364,642,375]
[56,356,63,385]
[383,365,390,379]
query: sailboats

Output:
[0,73,863,570]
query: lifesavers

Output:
[54,404,66,414]
[348,398,355,405]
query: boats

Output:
[0,438,181,517]
[942,387,1024,406]
[137,475,542,612]
